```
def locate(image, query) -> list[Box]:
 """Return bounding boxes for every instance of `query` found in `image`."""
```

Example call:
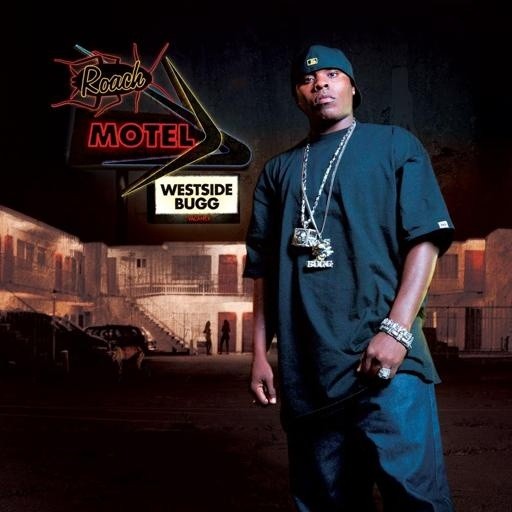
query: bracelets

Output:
[377,316,414,349]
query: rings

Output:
[379,368,392,380]
[252,399,257,404]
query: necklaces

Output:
[288,123,357,268]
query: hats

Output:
[292,45,360,109]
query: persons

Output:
[219,320,230,354]
[202,321,212,355]
[241,44,456,511]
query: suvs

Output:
[0,309,114,369]
[84,325,157,352]
